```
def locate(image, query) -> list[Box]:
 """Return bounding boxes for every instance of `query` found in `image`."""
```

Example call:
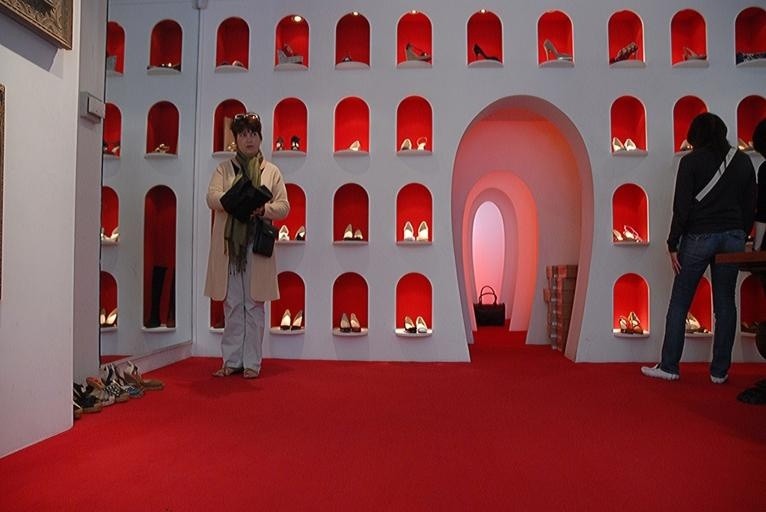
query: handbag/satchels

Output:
[220,174,273,224]
[472,302,505,326]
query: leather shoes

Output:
[278,225,305,241]
[344,223,363,240]
[400,138,411,149]
[279,308,303,330]
[741,321,760,333]
[146,63,180,70]
[101,308,117,326]
[101,139,121,154]
[339,312,361,332]
[101,226,118,241]
[233,59,243,67]
[342,56,351,62]
[348,140,361,151]
[404,220,429,241]
[611,136,640,151]
[737,136,752,151]
[619,311,643,334]
[686,311,709,335]
[679,139,694,150]
[404,315,427,333]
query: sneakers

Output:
[710,374,729,384]
[640,362,680,380]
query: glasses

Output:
[234,113,260,124]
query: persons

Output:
[204,111,291,380]
[736,118,766,406]
[638,110,756,385]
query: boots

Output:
[150,265,175,327]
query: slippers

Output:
[148,144,171,154]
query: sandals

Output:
[243,368,258,378]
[276,136,300,151]
[212,368,242,377]
[413,136,427,151]
[613,225,643,242]
[72,360,164,419]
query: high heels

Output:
[543,38,573,60]
[406,42,431,60]
[277,43,304,64]
[611,42,638,62]
[684,48,705,59]
[474,43,499,60]
